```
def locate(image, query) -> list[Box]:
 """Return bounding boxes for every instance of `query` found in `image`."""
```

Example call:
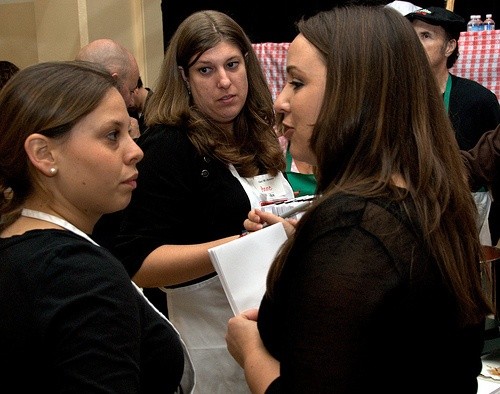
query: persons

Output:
[0,7,500,394]
[225,5,493,394]
[0,59,196,394]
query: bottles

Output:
[484,14,495,30]
[477,15,483,31]
[467,15,478,31]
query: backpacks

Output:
[352,179,485,394]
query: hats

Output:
[404,6,467,32]
